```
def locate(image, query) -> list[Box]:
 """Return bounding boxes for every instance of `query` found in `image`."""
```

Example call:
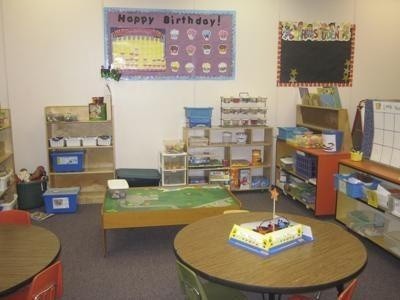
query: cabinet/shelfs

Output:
[45,103,115,205]
[0,110,18,211]
[162,104,400,258]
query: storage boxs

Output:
[50,151,86,172]
[42,186,81,214]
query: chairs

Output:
[175,260,248,300]
[0,210,31,224]
[287,277,359,300]
[5,260,62,300]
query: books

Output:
[187,152,231,190]
[279,156,294,170]
[188,135,210,148]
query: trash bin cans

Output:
[17,183,42,210]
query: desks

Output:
[100,184,242,258]
[172,211,369,300]
[0,223,63,298]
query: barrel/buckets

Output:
[322,130,343,152]
[16,178,43,210]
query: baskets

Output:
[49,139,64,147]
[295,154,317,178]
[98,138,112,145]
